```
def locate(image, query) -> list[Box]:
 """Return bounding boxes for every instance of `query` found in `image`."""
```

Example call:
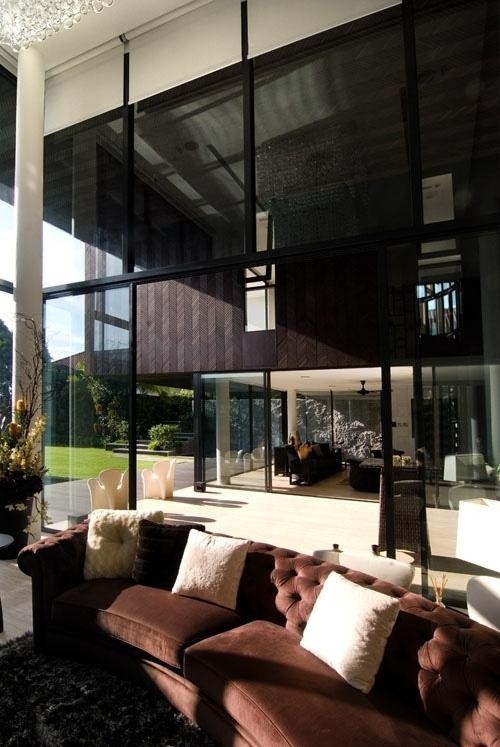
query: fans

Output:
[338,381,393,395]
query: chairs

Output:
[141,455,177,502]
[87,465,130,512]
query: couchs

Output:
[16,516,500,747]
[273,435,429,492]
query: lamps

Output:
[0,0,113,56]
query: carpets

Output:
[1,629,225,747]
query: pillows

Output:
[127,519,204,589]
[81,502,166,582]
[168,526,252,612]
[299,570,402,697]
